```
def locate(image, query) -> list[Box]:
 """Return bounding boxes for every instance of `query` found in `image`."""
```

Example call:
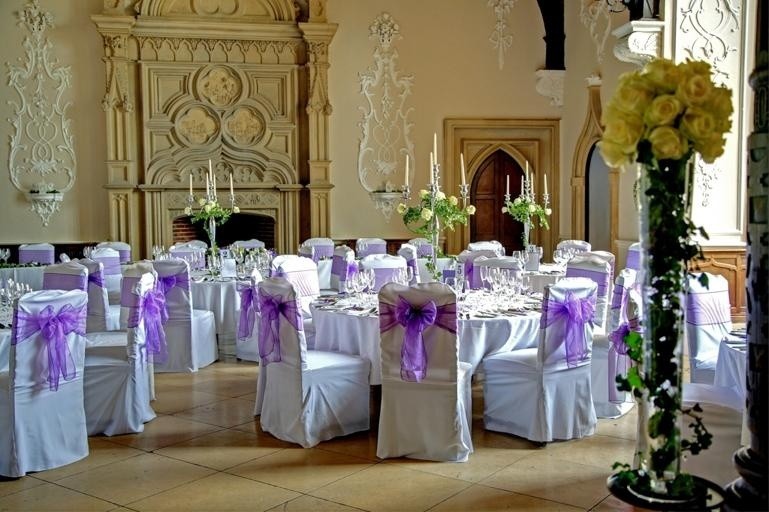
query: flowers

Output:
[501,198,553,231]
[396,190,477,239]
[184,198,240,227]
[597,56,734,169]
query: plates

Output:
[321,305,347,311]
[312,301,336,307]
[339,309,371,315]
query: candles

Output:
[520,174,524,197]
[525,159,529,182]
[229,172,233,196]
[543,173,547,193]
[530,172,534,193]
[189,173,192,196]
[213,173,216,197]
[209,158,212,182]
[433,133,438,165]
[206,172,209,196]
[460,152,465,185]
[405,154,409,186]
[506,174,510,194]
[429,152,433,184]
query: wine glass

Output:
[216,255,227,281]
[207,256,216,284]
[345,268,376,305]
[513,251,521,262]
[82,246,97,258]
[458,280,470,313]
[445,277,458,294]
[0,277,33,329]
[1,248,11,264]
[552,247,583,273]
[183,250,203,273]
[392,266,414,286]
[488,267,530,310]
[522,250,529,272]
[536,246,543,266]
[226,243,271,281]
[355,240,370,258]
[479,265,488,290]
[413,241,421,259]
[151,244,171,261]
